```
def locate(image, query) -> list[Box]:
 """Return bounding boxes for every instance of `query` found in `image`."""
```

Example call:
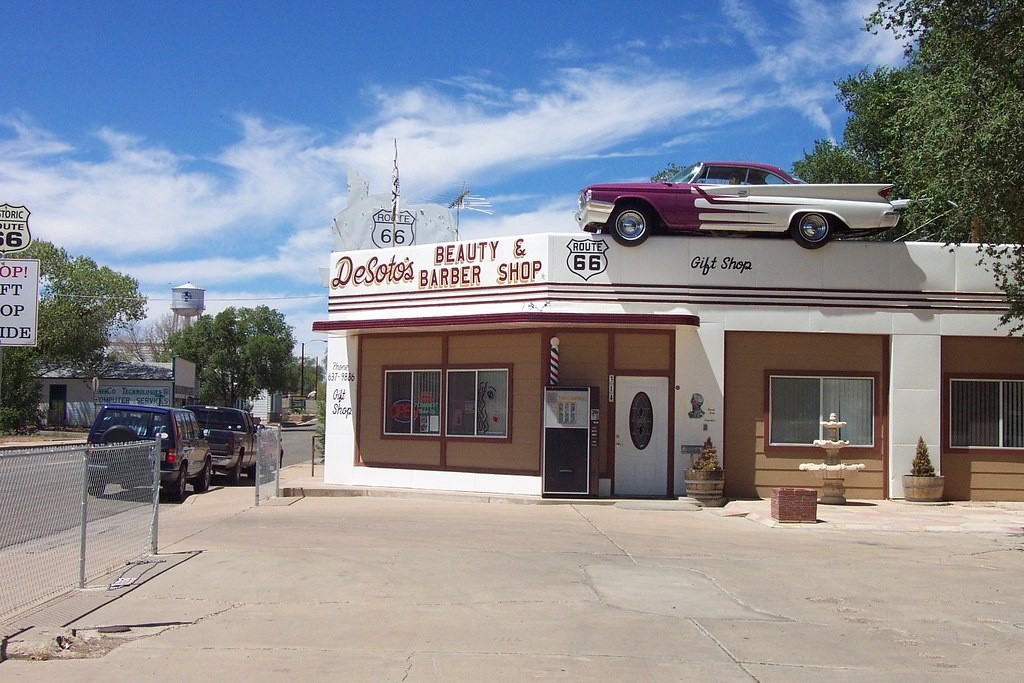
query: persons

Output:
[728,173,740,184]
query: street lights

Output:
[300,339,328,409]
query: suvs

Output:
[83,404,214,502]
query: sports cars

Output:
[578,159,914,250]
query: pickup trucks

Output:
[180,404,286,488]
